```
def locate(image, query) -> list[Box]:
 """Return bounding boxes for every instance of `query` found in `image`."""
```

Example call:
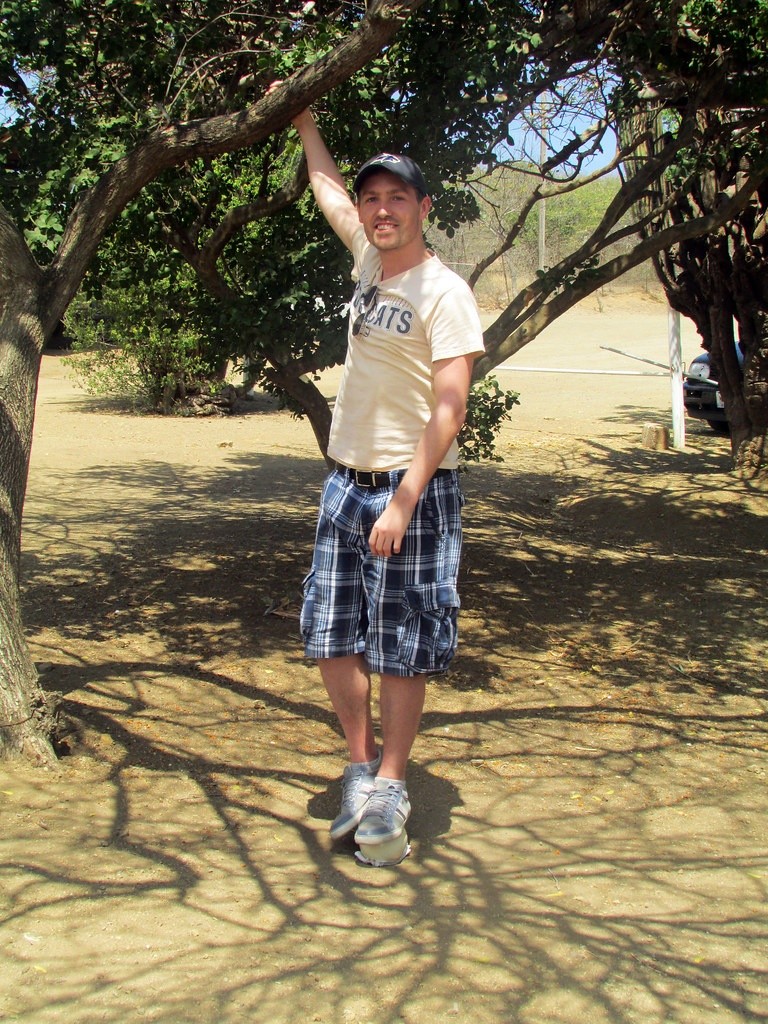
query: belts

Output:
[336,462,450,486]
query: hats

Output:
[353,152,428,194]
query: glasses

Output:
[351,284,377,336]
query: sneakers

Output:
[354,778,411,844]
[330,748,382,840]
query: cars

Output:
[682,340,744,433]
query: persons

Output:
[263,80,487,845]
